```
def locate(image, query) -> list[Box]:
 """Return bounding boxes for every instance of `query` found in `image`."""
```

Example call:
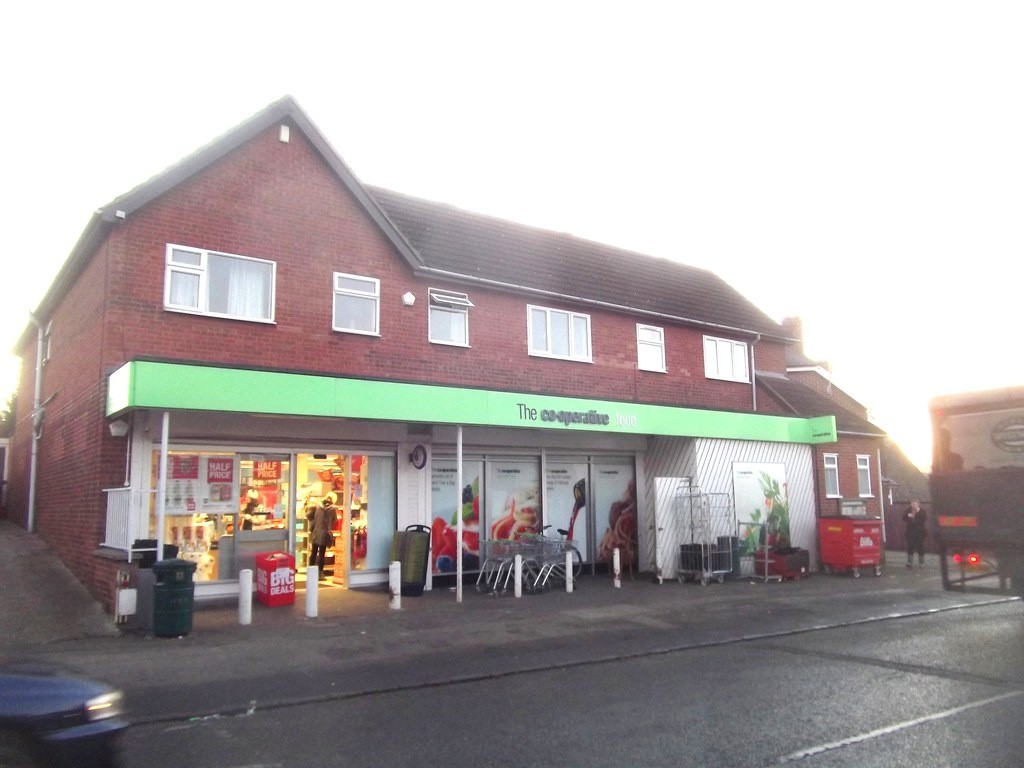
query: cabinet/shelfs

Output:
[331,471,361,511]
[296,516,341,572]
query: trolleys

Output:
[474,537,580,597]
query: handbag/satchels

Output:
[326,532,334,548]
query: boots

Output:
[918,555,924,568]
[907,554,913,567]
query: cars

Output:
[1,659,129,768]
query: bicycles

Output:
[528,525,583,579]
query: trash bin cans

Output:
[150,558,197,639]
[717,536,741,577]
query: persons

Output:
[241,496,260,530]
[305,492,338,581]
[902,499,927,569]
